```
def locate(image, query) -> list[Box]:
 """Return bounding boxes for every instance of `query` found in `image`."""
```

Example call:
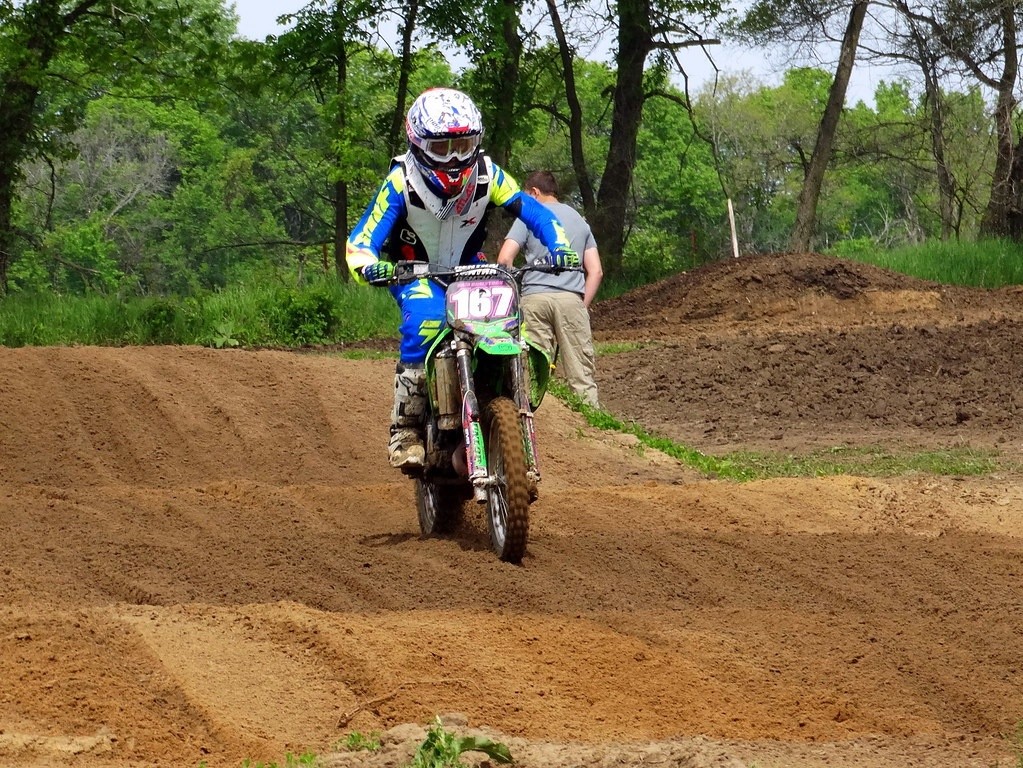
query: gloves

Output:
[364,261,394,286]
[550,244,582,277]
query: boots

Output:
[387,363,429,468]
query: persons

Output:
[345,87,581,469]
[497,171,603,409]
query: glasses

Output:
[424,134,481,164]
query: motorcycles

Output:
[362,258,587,567]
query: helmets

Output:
[405,88,485,201]
